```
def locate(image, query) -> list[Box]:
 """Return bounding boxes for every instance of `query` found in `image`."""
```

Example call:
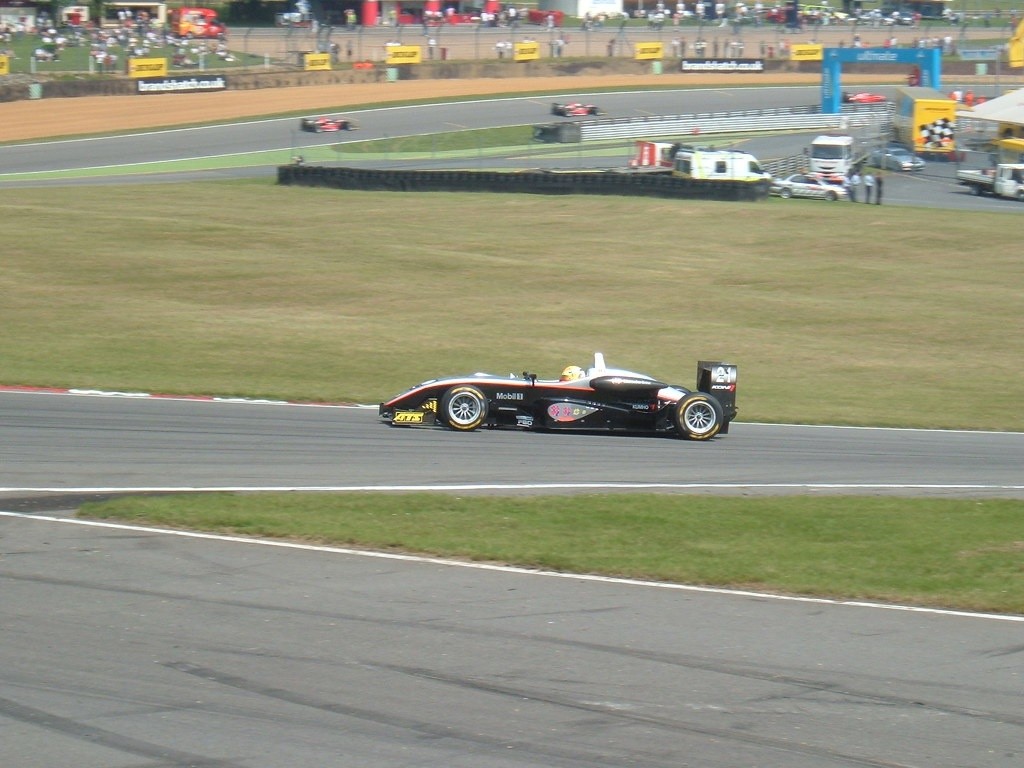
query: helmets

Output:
[559,365,586,382]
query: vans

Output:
[671,149,772,181]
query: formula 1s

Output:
[551,102,607,117]
[300,118,360,133]
[375,353,739,441]
[842,93,887,102]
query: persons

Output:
[953,87,986,106]
[292,155,304,167]
[290,0,571,64]
[560,366,585,382]
[581,0,1018,57]
[843,172,862,203]
[906,65,920,87]
[864,172,883,205]
[0,10,234,71]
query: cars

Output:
[769,173,848,201]
[873,147,926,172]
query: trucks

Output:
[803,134,870,180]
[956,164,1024,200]
[893,86,957,161]
